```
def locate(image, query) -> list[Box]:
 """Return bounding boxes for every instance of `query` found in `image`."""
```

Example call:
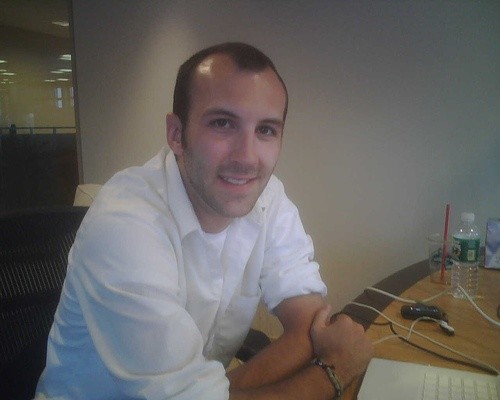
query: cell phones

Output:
[402,304,446,322]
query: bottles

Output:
[449,212,480,301]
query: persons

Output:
[30,41,371,400]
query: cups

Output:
[425,234,455,285]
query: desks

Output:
[337,246,500,400]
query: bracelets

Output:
[310,358,345,400]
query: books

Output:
[356,356,500,400]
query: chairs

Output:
[0,207,270,400]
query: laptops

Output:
[357,358,500,400]
